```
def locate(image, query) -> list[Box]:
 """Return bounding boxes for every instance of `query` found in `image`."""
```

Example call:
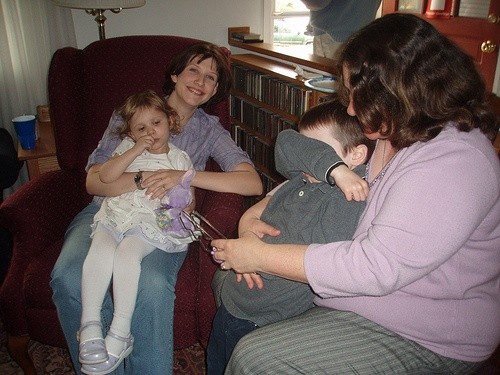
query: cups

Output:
[12,115,38,150]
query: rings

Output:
[163,185,167,191]
[220,263,227,269]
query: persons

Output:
[75,89,196,375]
[48,42,262,375]
[302,0,382,59]
[211,14,499,374]
[202,100,378,375]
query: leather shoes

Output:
[80,330,134,375]
[76,320,109,364]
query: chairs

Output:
[0,34,245,375]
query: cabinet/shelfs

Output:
[232,53,327,199]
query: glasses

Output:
[179,210,228,255]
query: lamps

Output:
[50,0,145,40]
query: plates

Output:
[303,77,339,93]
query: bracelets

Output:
[181,169,196,188]
[133,169,146,190]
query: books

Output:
[229,66,313,211]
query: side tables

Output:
[18,121,59,181]
